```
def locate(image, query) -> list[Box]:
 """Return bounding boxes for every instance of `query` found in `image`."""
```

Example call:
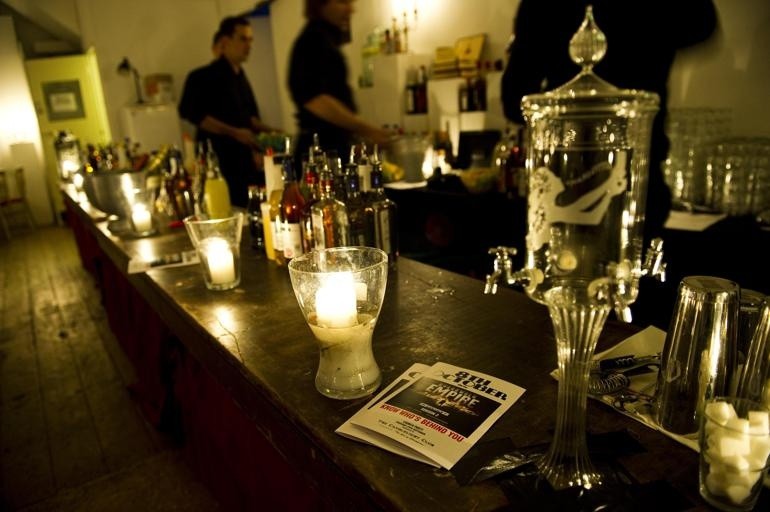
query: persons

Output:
[500,0,720,234]
[176,15,291,213]
[286,0,392,158]
[192,33,224,155]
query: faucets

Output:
[482,245,531,297]
[638,237,670,284]
[596,261,635,327]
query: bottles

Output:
[358,9,420,88]
[398,127,456,158]
[247,127,397,269]
[206,138,231,217]
[81,134,204,218]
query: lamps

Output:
[116,57,144,103]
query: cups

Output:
[287,246,389,400]
[724,289,769,419]
[183,209,245,292]
[648,275,741,437]
[458,77,486,111]
[403,83,427,114]
[698,397,770,512]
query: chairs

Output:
[0,168,39,243]
